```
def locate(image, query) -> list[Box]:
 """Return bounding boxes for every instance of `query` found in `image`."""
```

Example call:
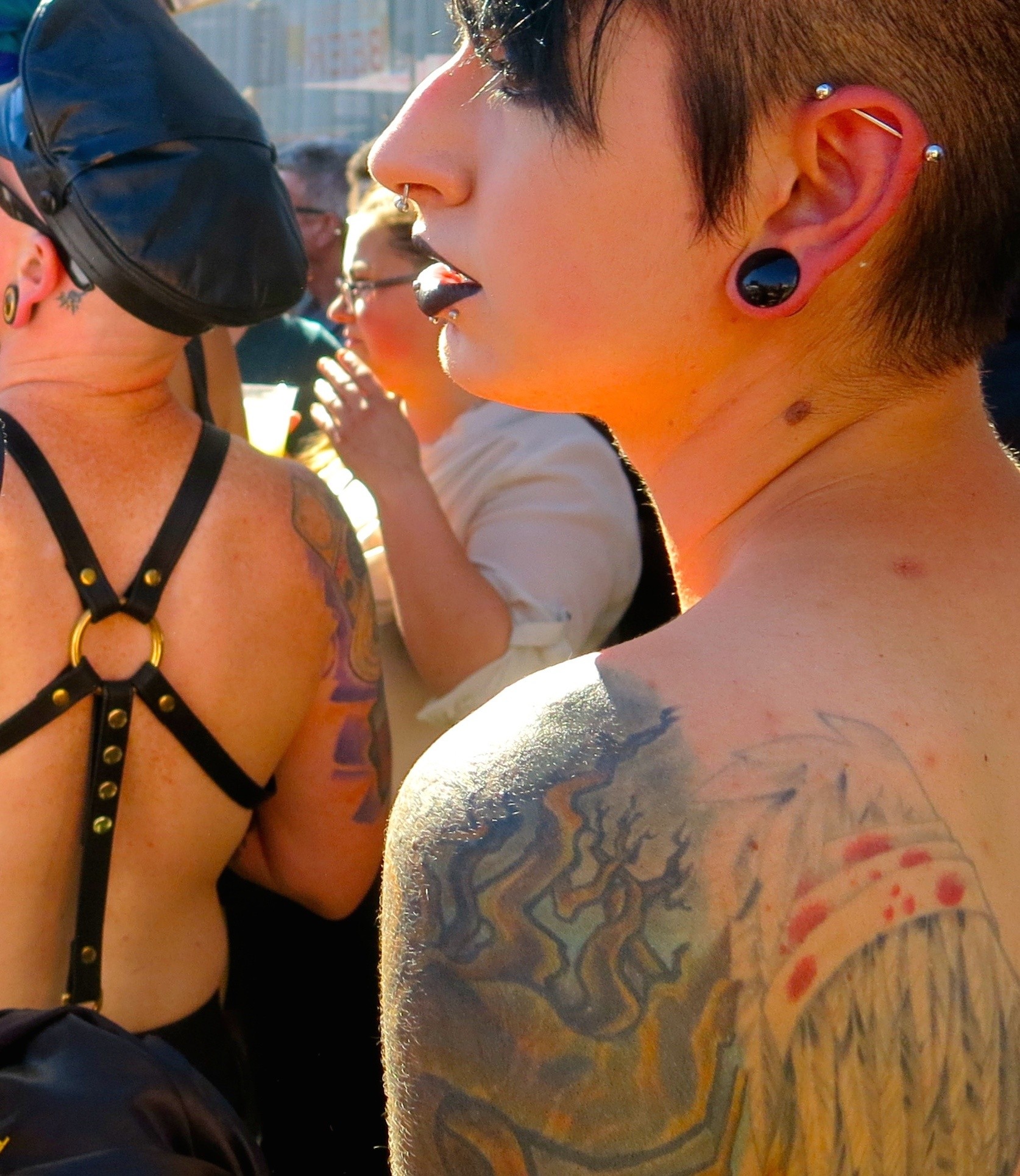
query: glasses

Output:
[335,265,430,312]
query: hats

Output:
[7,0,313,341]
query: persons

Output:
[336,134,390,208]
[359,0,1020,1175]
[228,311,344,469]
[1,0,398,1176]
[265,135,349,348]
[165,322,252,446]
[254,183,645,945]
